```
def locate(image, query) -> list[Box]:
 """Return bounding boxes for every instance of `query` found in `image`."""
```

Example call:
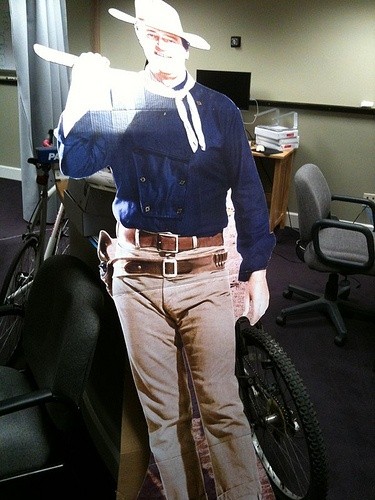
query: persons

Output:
[57,0,277,500]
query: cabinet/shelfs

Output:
[251,146,295,233]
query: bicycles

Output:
[0,128,331,500]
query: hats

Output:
[108,0,213,53]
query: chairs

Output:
[0,253,102,484]
[276,164,375,347]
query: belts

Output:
[123,248,227,277]
[117,222,225,254]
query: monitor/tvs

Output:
[196,70,250,110]
